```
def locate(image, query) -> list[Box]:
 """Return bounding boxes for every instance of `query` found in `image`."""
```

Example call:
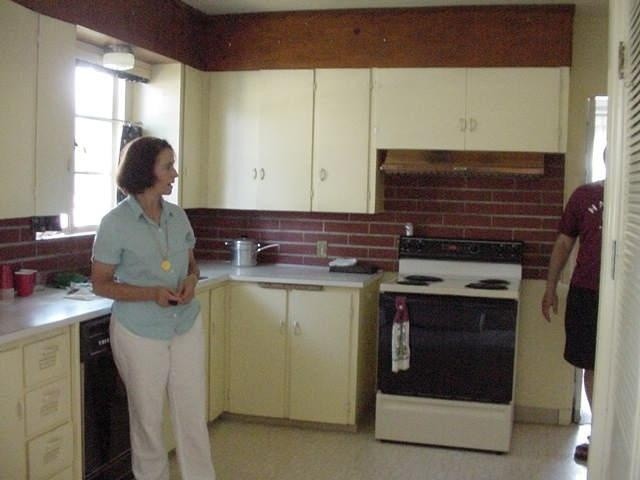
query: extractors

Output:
[379,149,547,179]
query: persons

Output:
[541,147,607,461]
[91,136,218,480]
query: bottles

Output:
[404,222,413,236]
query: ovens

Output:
[79,316,137,479]
[377,294,519,406]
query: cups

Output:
[15,268,38,297]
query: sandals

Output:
[574,435,591,460]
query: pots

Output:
[228,235,281,268]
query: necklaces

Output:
[146,219,170,271]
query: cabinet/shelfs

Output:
[0,319,82,480]
[0,0,77,222]
[373,67,570,150]
[226,274,383,426]
[204,67,313,211]
[161,275,230,456]
[135,64,205,209]
[311,64,383,213]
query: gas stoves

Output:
[376,274,523,301]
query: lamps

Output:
[97,41,139,73]
